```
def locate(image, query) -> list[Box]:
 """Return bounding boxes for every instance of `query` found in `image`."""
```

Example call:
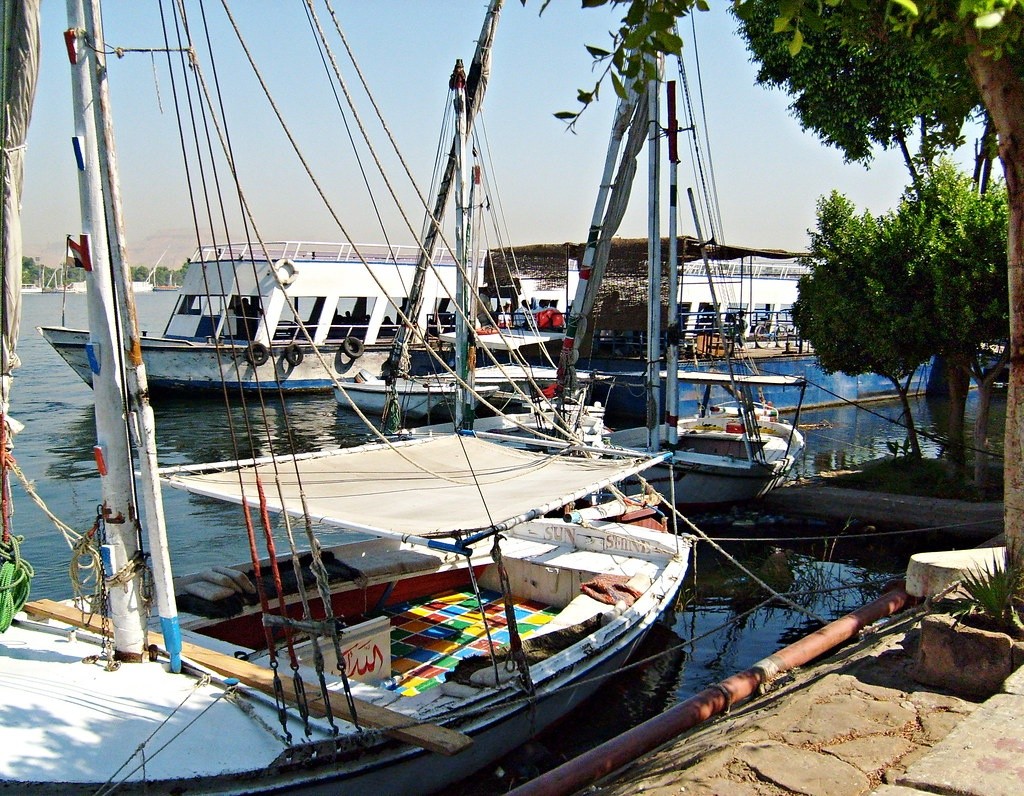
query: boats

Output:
[650,367,808,480]
[30,235,831,398]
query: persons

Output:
[600,291,620,316]
[236,298,394,338]
[513,299,566,329]
[731,310,748,351]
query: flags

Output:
[67,237,84,268]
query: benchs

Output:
[146,536,669,721]
[629,429,790,463]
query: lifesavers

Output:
[474,326,499,335]
[342,335,365,360]
[244,342,270,367]
[285,343,304,367]
[271,256,299,286]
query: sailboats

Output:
[2,1,695,794]
[328,2,608,423]
